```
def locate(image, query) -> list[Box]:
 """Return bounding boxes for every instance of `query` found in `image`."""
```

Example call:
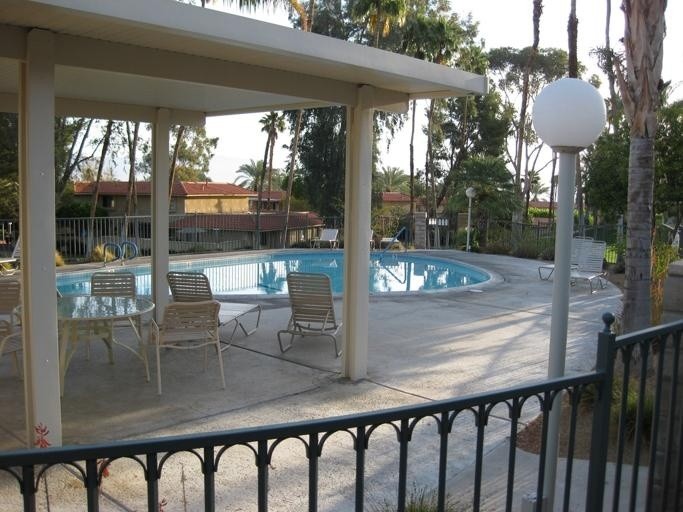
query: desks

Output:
[12,295,154,399]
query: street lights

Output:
[466,187,476,252]
[532,78,607,512]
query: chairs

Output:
[165,270,261,353]
[570,240,608,294]
[276,271,342,357]
[0,233,21,376]
[148,300,226,395]
[537,236,594,280]
[84,271,141,359]
[310,228,339,249]
